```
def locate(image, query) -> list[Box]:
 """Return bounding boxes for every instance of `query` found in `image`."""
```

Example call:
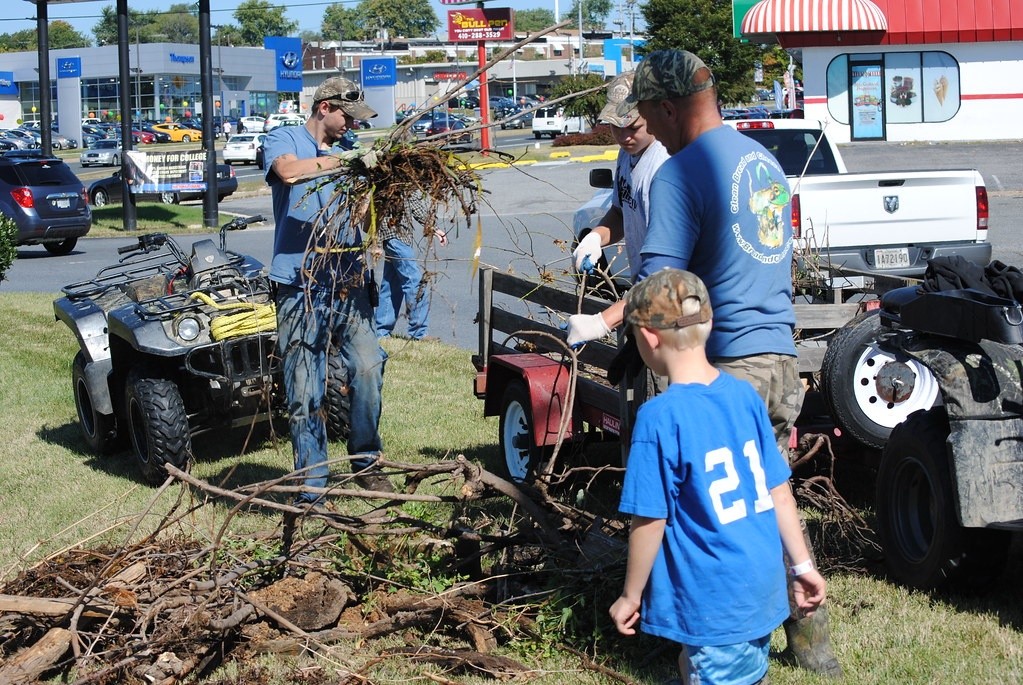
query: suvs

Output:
[51,214,352,488]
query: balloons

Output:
[0,100,220,125]
[259,101,308,117]
[397,88,526,117]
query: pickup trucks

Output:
[572,118,993,302]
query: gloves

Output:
[341,151,382,172]
[571,233,603,275]
[558,313,611,350]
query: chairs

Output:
[776,140,808,167]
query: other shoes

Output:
[777,609,844,677]
[356,469,395,495]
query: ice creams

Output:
[934,75,948,106]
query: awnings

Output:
[739,0,887,43]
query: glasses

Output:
[317,90,367,104]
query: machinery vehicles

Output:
[465,257,1023,592]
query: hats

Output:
[624,266,713,330]
[596,73,643,129]
[616,48,718,118]
[313,76,378,120]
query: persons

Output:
[608,264,828,685]
[263,75,397,519]
[105,125,117,140]
[222,119,232,142]
[566,48,843,685]
[572,71,675,285]
[236,117,245,134]
[371,176,449,342]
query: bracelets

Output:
[788,559,815,577]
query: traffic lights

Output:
[507,88,513,96]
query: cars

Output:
[86,161,239,205]
[721,106,804,120]
[425,119,473,144]
[79,139,139,168]
[531,108,585,140]
[0,120,78,154]
[223,133,268,165]
[280,119,305,126]
[0,148,92,255]
[263,114,307,132]
[237,116,266,133]
[351,95,549,133]
[753,87,804,109]
[81,117,237,149]
[256,143,264,170]
